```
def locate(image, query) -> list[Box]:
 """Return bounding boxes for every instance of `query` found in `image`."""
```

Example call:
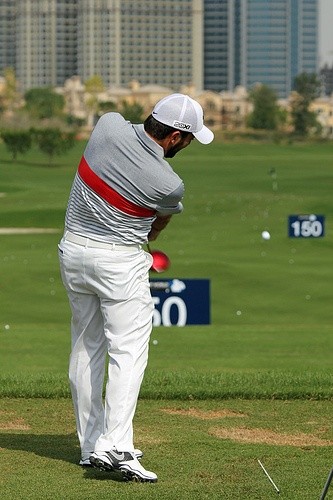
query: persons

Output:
[57,93,214,482]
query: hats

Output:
[152,93,215,145]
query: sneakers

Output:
[79,448,158,481]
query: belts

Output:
[63,229,141,251]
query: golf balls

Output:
[261,231,271,240]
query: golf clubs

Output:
[147,243,170,273]
